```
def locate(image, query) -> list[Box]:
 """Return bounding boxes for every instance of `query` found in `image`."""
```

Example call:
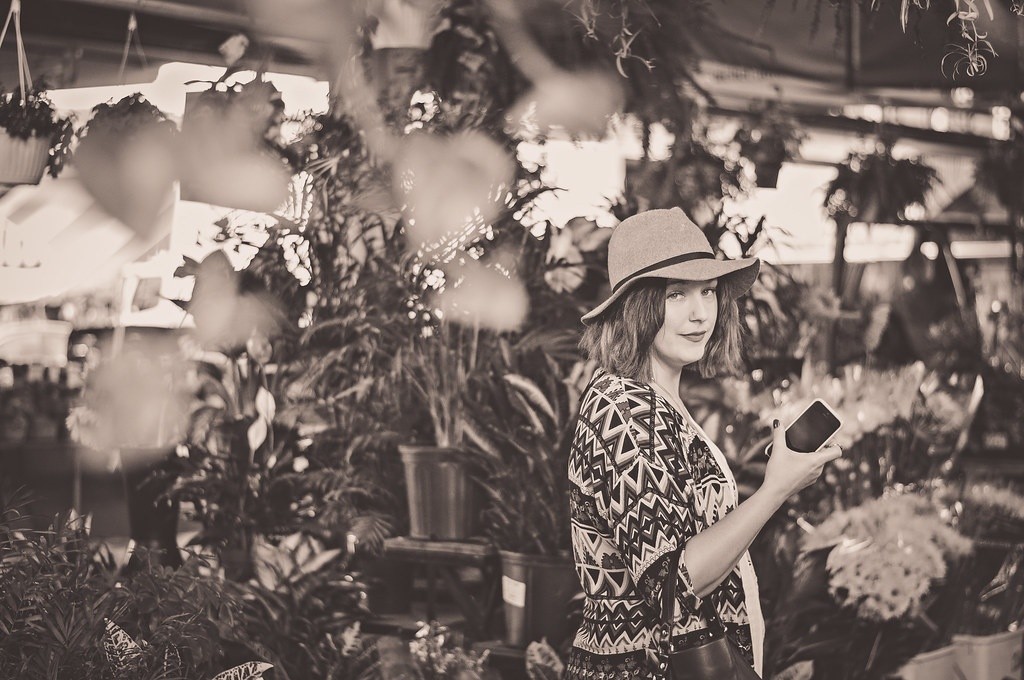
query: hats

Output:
[575,203,762,325]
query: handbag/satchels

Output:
[655,546,762,680]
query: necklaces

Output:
[652,378,696,430]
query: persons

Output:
[567,206,842,679]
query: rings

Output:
[832,444,838,446]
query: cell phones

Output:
[764,398,843,460]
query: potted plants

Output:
[823,151,946,229]
[0,70,78,188]
[396,302,505,547]
[179,33,294,212]
[73,92,184,228]
[436,351,586,654]
[737,97,807,190]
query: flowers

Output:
[754,350,930,456]
[795,490,978,621]
